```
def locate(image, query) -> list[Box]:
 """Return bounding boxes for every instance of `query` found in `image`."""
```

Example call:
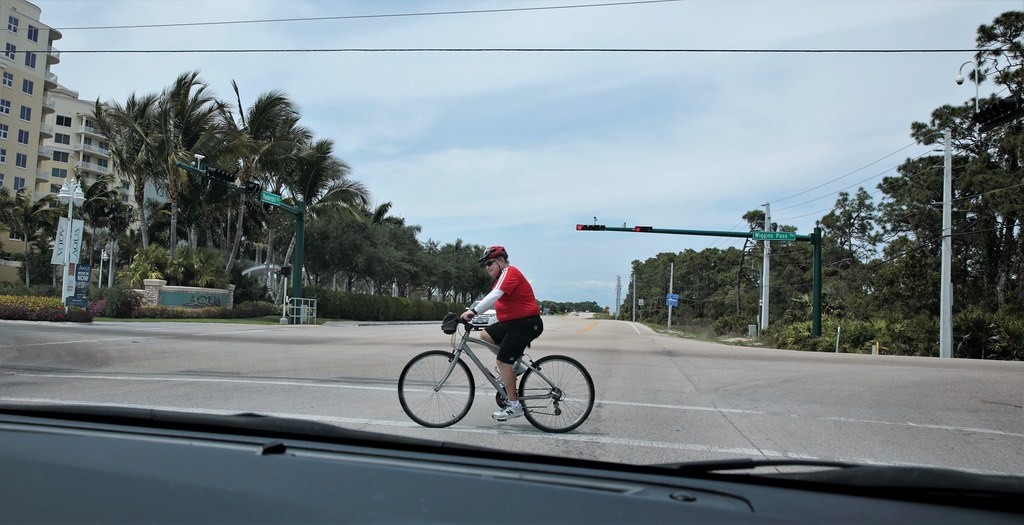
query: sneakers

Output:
[496,361,526,383]
[492,404,524,420]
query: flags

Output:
[69,218,84,264]
[50,216,68,266]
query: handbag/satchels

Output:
[441,311,458,334]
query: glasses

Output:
[484,259,498,267]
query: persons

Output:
[460,246,544,420]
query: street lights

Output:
[56,177,87,308]
[957,60,980,114]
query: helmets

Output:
[477,246,508,262]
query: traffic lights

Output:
[969,94,1018,124]
[576,225,606,231]
[207,167,235,183]
[246,179,264,195]
[634,226,654,233]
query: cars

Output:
[458,301,497,331]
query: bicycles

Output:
[397,308,595,433]
